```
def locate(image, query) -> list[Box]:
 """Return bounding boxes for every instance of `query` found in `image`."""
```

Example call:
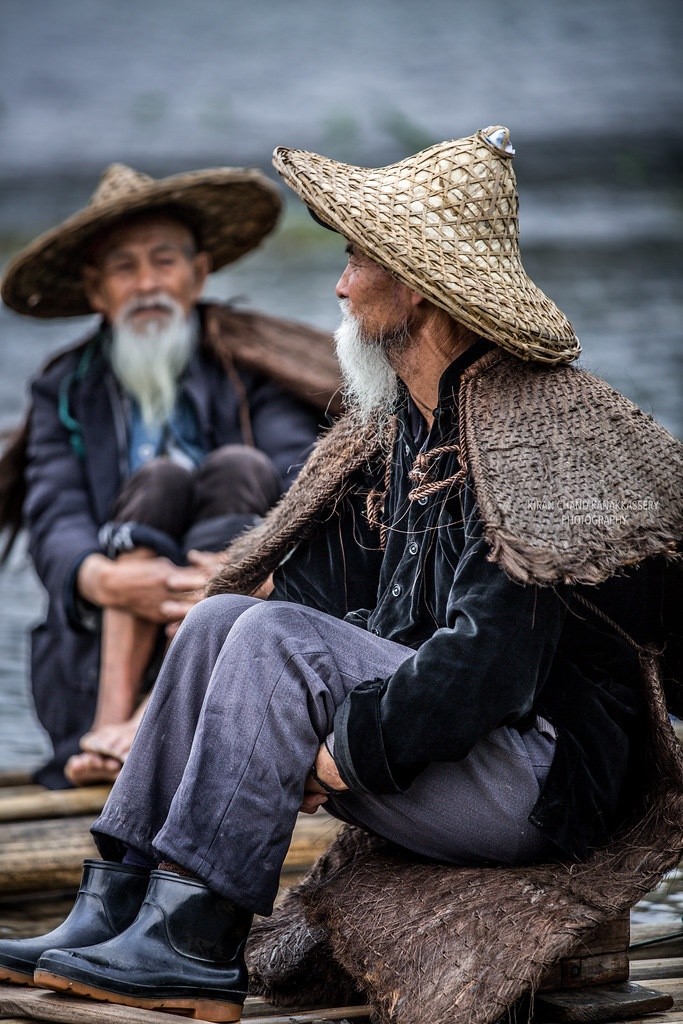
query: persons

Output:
[0,123,683,1023]
[0,162,343,791]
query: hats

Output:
[270,125,582,367]
[0,163,284,319]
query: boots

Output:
[0,859,154,989]
[34,869,254,1023]
